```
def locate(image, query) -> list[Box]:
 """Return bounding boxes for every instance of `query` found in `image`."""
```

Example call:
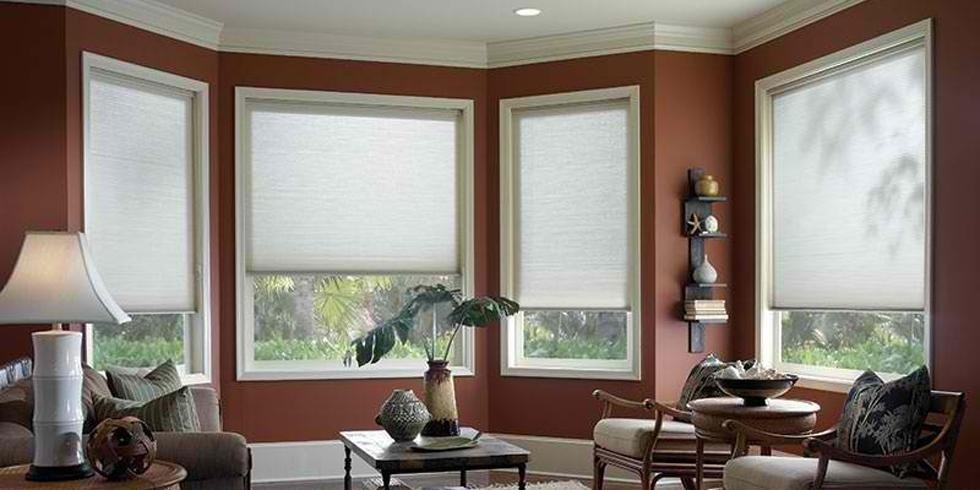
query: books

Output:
[685,299,729,322]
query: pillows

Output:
[91,359,202,434]
[837,364,931,479]
[672,352,757,423]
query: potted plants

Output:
[343,282,519,437]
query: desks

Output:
[0,459,188,490]
[685,397,821,490]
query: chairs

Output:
[723,390,966,490]
[592,389,750,490]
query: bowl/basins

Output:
[714,374,798,405]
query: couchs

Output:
[0,361,252,490]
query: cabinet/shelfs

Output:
[676,167,729,354]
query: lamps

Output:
[1,230,133,482]
